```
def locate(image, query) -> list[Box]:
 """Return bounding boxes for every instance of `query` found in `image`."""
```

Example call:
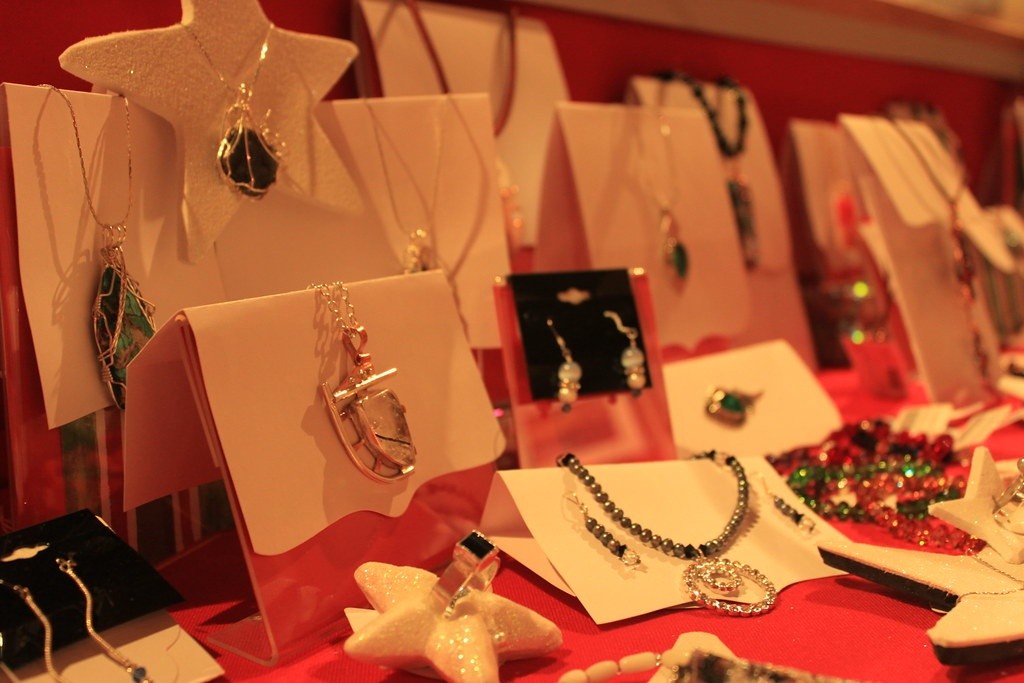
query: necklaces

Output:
[363,95,448,274]
[620,106,688,280]
[957,548,1024,599]
[552,446,779,618]
[178,22,291,202]
[403,0,523,245]
[308,279,418,486]
[657,69,760,272]
[766,419,987,557]
[39,82,157,409]
[557,648,873,683]
[815,105,996,400]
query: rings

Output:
[428,529,501,618]
